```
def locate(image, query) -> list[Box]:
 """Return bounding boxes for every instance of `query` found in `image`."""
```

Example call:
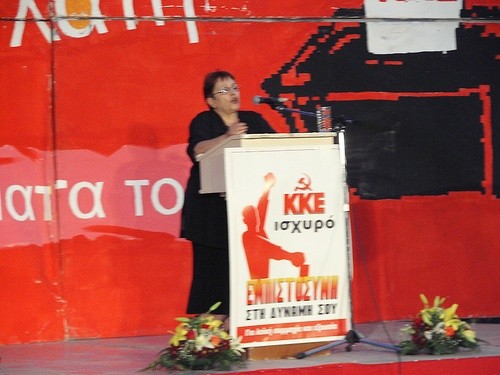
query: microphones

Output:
[252,95,289,104]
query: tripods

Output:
[269,102,416,359]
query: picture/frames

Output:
[224,145,353,350]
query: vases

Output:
[182,356,219,370]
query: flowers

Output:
[395,294,489,355]
[138,302,247,371]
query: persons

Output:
[178,70,281,331]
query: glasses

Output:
[212,84,240,97]
